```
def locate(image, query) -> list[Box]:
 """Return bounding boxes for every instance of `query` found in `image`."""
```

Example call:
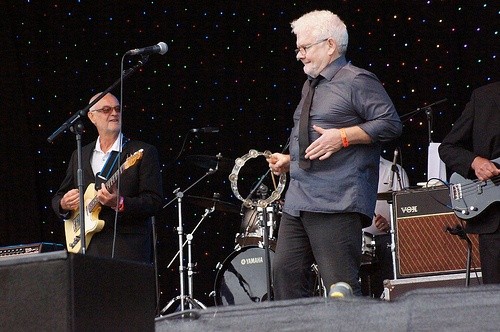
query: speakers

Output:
[391,186,481,278]
[0,249,157,332]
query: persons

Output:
[266,9,403,302]
[52,92,164,263]
[438,82,500,286]
[362,156,408,297]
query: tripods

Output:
[160,168,329,317]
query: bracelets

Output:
[113,196,124,209]
[340,127,349,148]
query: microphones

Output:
[388,149,398,191]
[126,41,168,55]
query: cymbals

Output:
[186,155,235,173]
[182,195,240,213]
[376,190,399,202]
[229,149,287,208]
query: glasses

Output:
[91,105,121,114]
[295,39,327,55]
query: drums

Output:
[361,230,375,266]
[236,200,284,251]
[214,244,276,306]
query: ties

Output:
[298,75,324,170]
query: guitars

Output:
[64,149,144,255]
[449,156,500,220]
